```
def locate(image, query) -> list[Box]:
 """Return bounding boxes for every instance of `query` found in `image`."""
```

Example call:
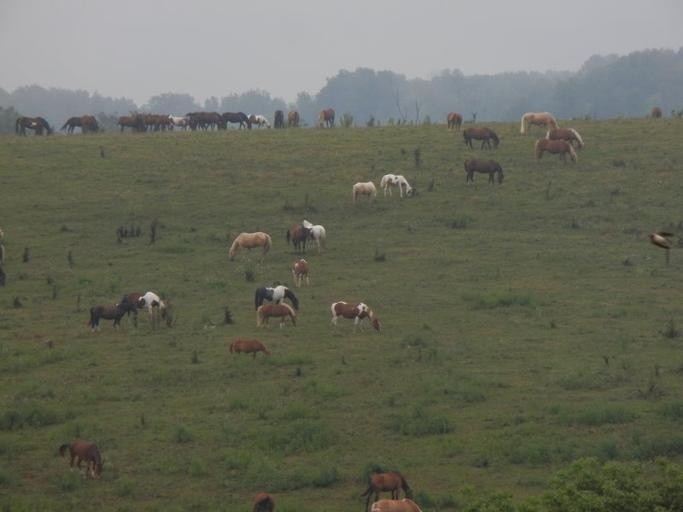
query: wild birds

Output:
[645,229,674,250]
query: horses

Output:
[330,301,380,333]
[59,115,99,135]
[361,466,420,511]
[252,491,274,511]
[118,111,299,135]
[286,218,327,253]
[446,111,463,130]
[518,111,583,165]
[463,158,504,187]
[227,231,271,261]
[378,172,412,200]
[227,338,270,361]
[463,127,500,151]
[14,116,52,137]
[290,259,311,288]
[254,285,299,329]
[87,291,166,333]
[317,107,334,129]
[59,437,106,478]
[351,181,377,206]
